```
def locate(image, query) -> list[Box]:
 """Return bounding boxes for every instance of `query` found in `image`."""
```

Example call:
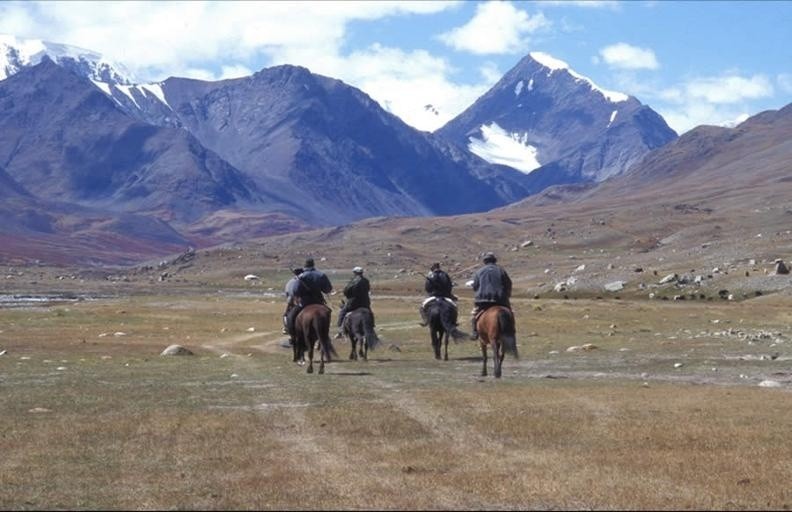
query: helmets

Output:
[353,267,364,274]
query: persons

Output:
[284,258,333,345]
[281,267,304,335]
[415,261,464,328]
[332,267,371,340]
[466,250,519,341]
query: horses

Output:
[291,302,339,375]
[426,295,470,362]
[475,303,520,379]
[341,306,381,363]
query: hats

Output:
[294,268,303,274]
[484,252,497,262]
[304,257,314,266]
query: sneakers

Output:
[334,333,342,339]
[469,332,478,340]
[289,336,296,346]
[282,327,287,334]
